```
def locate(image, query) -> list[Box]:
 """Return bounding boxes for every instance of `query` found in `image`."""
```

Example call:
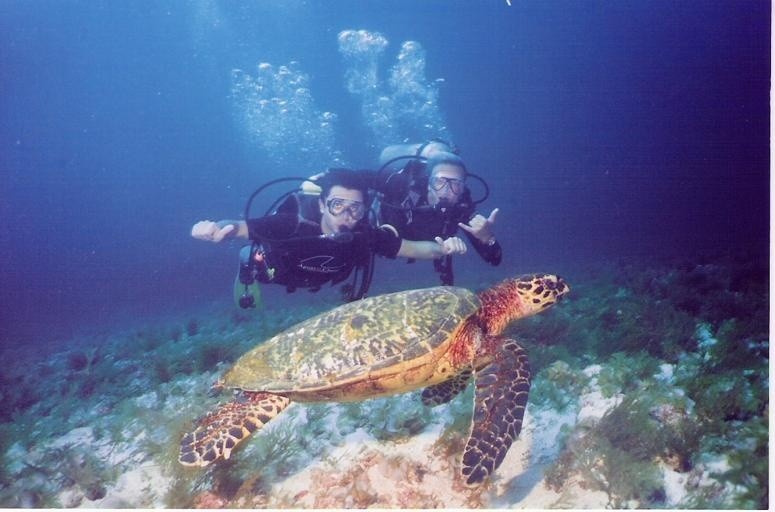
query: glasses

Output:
[327,198,367,221]
[428,176,465,195]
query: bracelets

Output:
[483,237,498,245]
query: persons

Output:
[349,138,503,286]
[190,167,468,314]
